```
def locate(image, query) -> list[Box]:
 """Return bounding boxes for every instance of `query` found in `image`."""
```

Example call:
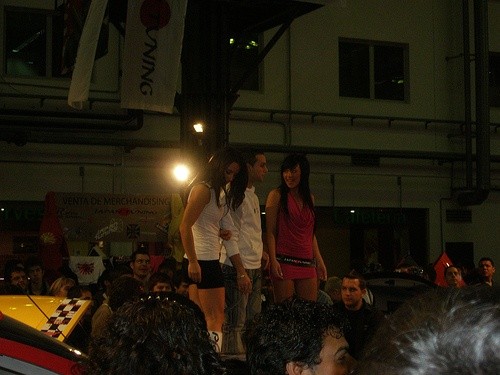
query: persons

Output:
[0,247,197,358]
[178,146,249,356]
[71,292,233,374]
[350,287,500,375]
[444,265,465,287]
[473,257,498,287]
[264,151,328,304]
[221,145,271,359]
[245,294,361,375]
[327,267,381,360]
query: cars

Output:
[0,294,97,375]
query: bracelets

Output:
[236,273,247,278]
